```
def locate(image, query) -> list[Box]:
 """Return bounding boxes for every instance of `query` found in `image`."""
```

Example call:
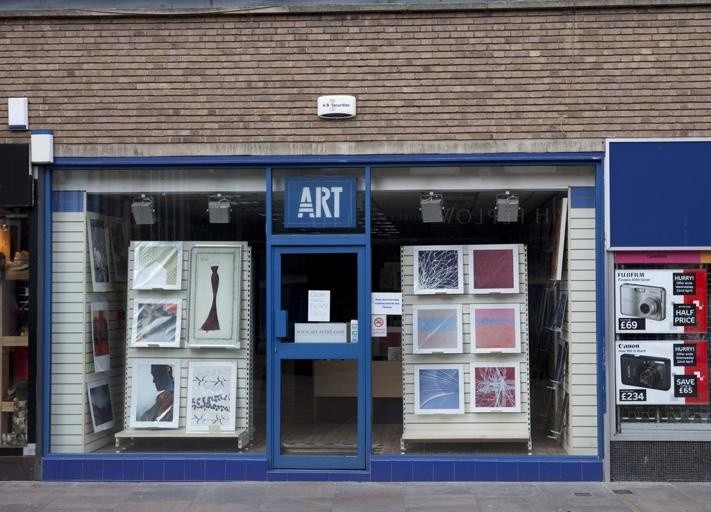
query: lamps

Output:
[131,194,158,226]
[494,191,520,223]
[206,194,233,225]
[419,193,445,224]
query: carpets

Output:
[281,442,385,455]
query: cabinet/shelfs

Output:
[0,226,30,451]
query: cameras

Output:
[620,283,667,321]
[620,354,671,391]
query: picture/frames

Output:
[412,245,521,415]
[129,240,243,432]
